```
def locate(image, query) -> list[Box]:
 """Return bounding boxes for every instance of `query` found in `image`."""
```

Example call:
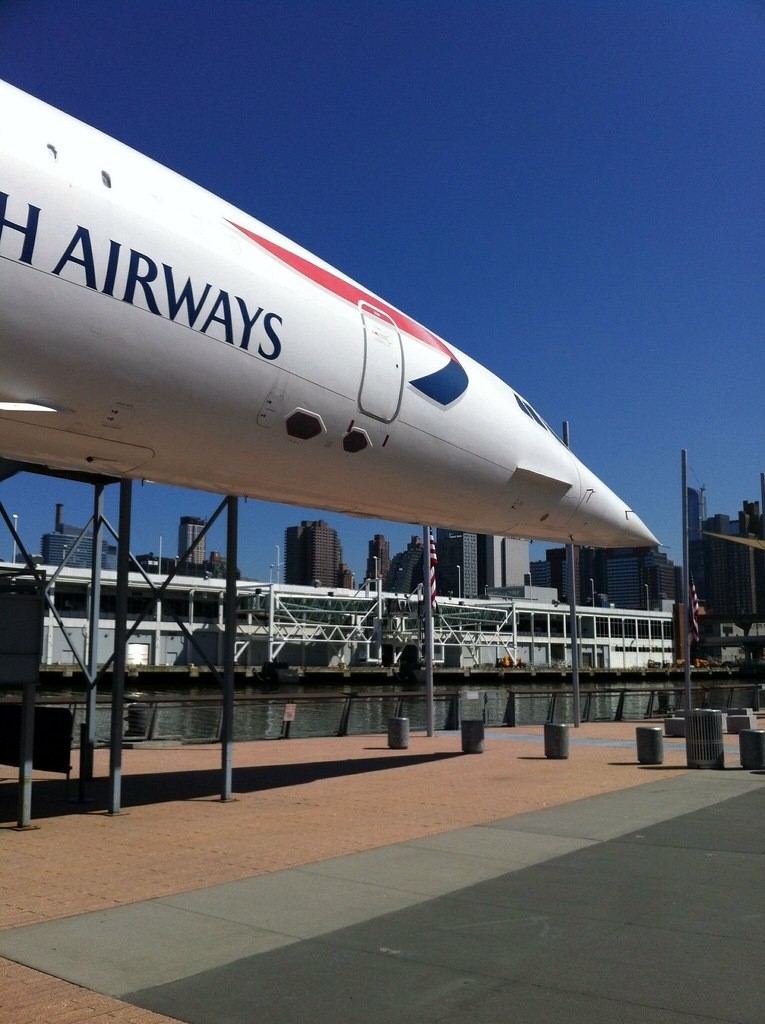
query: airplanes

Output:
[0,77,664,550]
